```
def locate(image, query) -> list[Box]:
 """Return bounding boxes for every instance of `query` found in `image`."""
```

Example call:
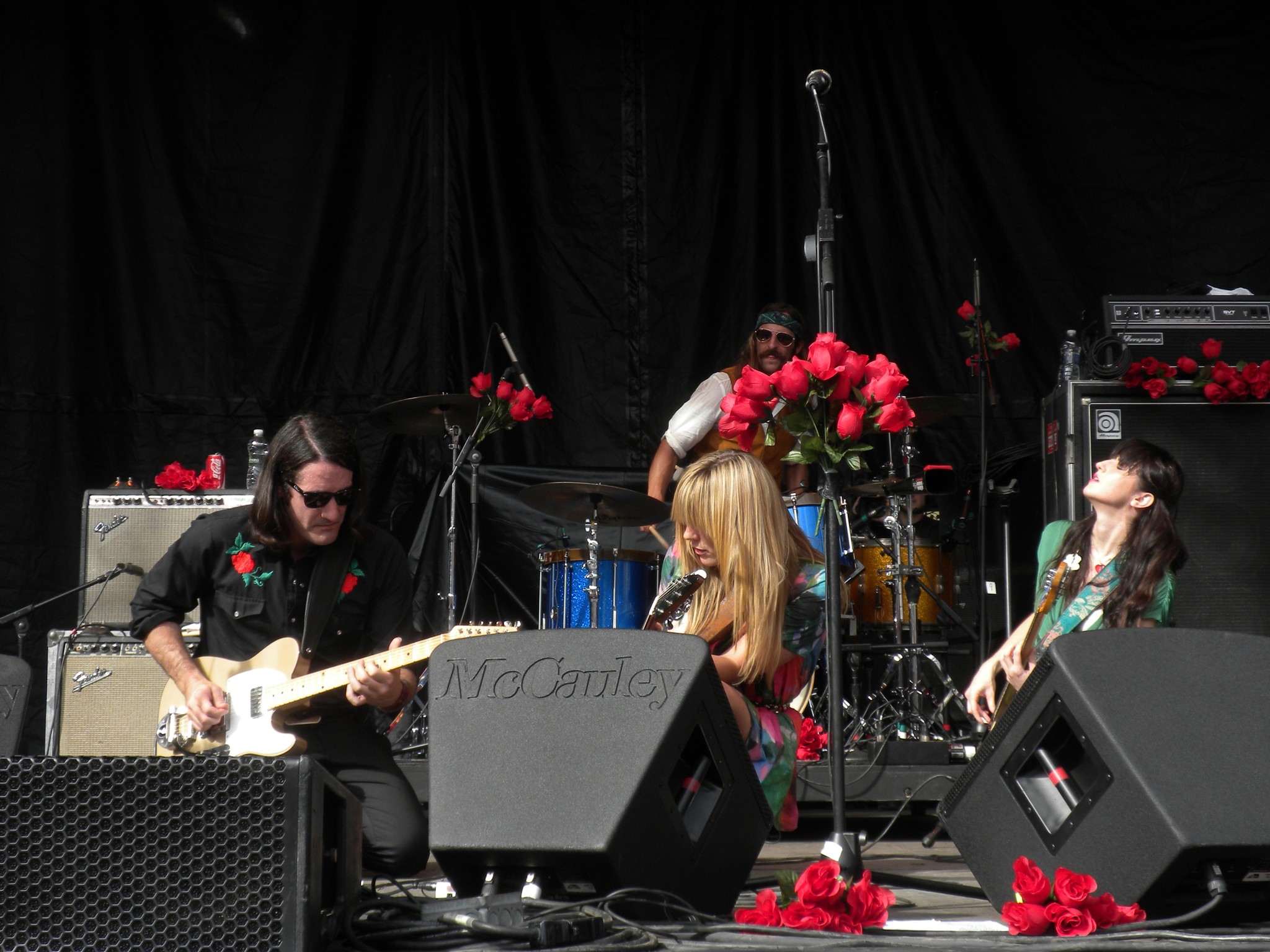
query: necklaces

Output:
[1090,536,1116,574]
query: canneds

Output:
[206,453,226,490]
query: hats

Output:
[757,310,805,343]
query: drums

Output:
[540,545,665,631]
[851,537,960,635]
[780,492,858,600]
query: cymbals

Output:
[369,390,494,442]
[849,477,928,496]
[519,479,672,528]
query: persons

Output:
[638,450,848,822]
[857,464,972,736]
[125,413,428,881]
[638,308,811,534]
[964,438,1186,727]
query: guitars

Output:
[153,618,524,763]
[644,569,707,631]
[988,552,1082,731]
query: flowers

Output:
[466,372,554,452]
[778,716,829,830]
[154,461,220,491]
[1120,356,1177,400]
[717,331,916,537]
[1177,339,1270,405]
[957,301,1021,399]
[734,860,896,936]
[1000,855,1146,937]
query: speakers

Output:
[52,636,201,755]
[0,752,364,952]
[936,628,1270,931]
[1043,382,1270,639]
[429,627,774,923]
[79,489,258,629]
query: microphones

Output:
[116,563,144,578]
[806,69,831,94]
[973,262,981,309]
[498,328,534,398]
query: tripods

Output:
[742,426,987,899]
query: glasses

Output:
[283,478,356,509]
[1110,449,1150,478]
[754,328,797,347]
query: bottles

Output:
[1058,329,1082,388]
[246,429,269,490]
